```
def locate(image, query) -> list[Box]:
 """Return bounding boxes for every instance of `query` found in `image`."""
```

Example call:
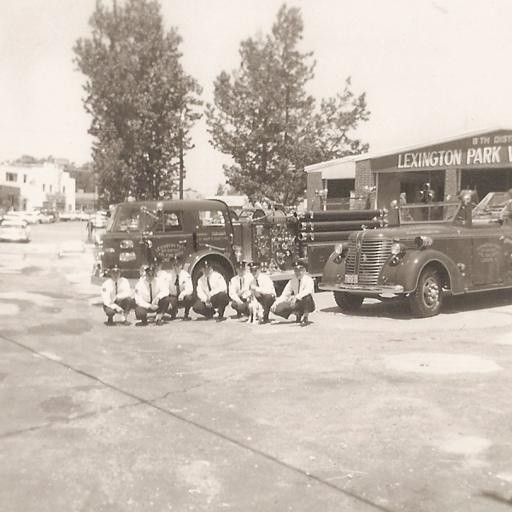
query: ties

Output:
[116,275,300,304]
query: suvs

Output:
[322,190,512,318]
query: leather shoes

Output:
[108,311,309,326]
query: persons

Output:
[192,260,229,322]
[87,221,93,238]
[101,264,134,326]
[166,255,193,321]
[248,261,277,325]
[228,260,254,318]
[268,260,316,327]
[134,266,169,326]
[152,255,169,283]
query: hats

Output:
[110,256,305,272]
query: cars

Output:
[0,203,110,245]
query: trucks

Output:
[90,200,387,299]
